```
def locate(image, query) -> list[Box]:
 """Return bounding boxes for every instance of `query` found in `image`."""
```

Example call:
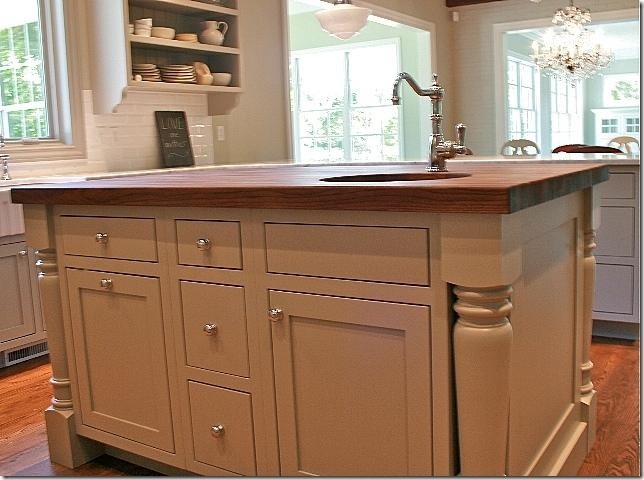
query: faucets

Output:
[390,71,467,172]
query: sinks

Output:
[319,173,472,183]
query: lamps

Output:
[313,0,373,39]
[528,0,614,89]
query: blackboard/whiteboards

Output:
[155,111,195,167]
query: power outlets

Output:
[217,126,224,141]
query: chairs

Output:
[500,140,541,156]
[552,145,624,153]
[608,136,639,153]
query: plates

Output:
[132,63,195,84]
[190,61,210,74]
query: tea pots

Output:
[197,20,228,45]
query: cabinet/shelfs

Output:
[86,0,244,116]
[590,107,640,153]
[52,206,186,470]
[251,210,430,476]
[164,207,268,477]
[592,166,641,323]
[1,232,47,352]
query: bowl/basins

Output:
[212,72,232,86]
[127,17,199,42]
[196,73,214,85]
